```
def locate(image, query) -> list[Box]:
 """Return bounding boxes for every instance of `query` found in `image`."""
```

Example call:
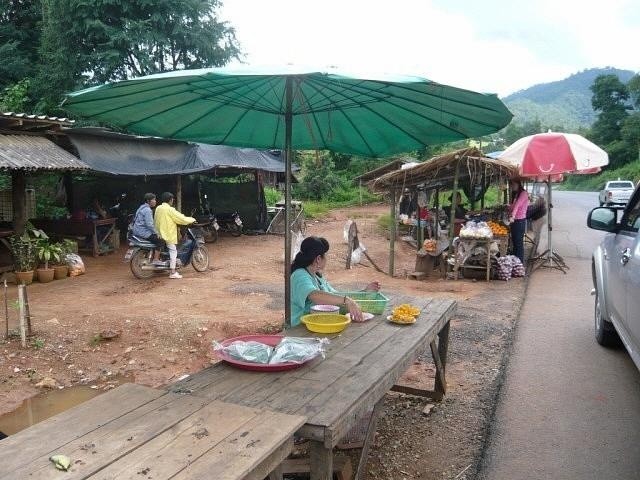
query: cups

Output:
[310,305,341,315]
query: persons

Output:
[448,192,466,223]
[132,193,166,265]
[509,180,529,263]
[154,192,196,279]
[291,237,380,327]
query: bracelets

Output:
[344,296,346,305]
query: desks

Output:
[154,287,462,480]
[27,216,120,257]
[0,376,312,480]
[491,234,511,258]
[454,236,498,284]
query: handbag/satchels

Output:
[526,205,538,219]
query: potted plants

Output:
[10,226,76,286]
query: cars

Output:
[589,178,640,371]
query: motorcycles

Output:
[184,206,219,245]
[108,193,135,238]
[124,209,209,281]
[201,194,244,238]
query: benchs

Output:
[414,234,451,279]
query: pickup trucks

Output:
[597,180,635,209]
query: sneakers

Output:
[168,271,183,279]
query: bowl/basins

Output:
[300,313,351,334]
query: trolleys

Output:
[265,204,308,237]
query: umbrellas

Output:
[529,167,602,248]
[56,67,515,331]
[498,129,609,266]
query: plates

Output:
[383,316,418,324]
[214,334,306,372]
[346,312,374,322]
[391,310,421,317]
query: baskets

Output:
[332,292,389,315]
